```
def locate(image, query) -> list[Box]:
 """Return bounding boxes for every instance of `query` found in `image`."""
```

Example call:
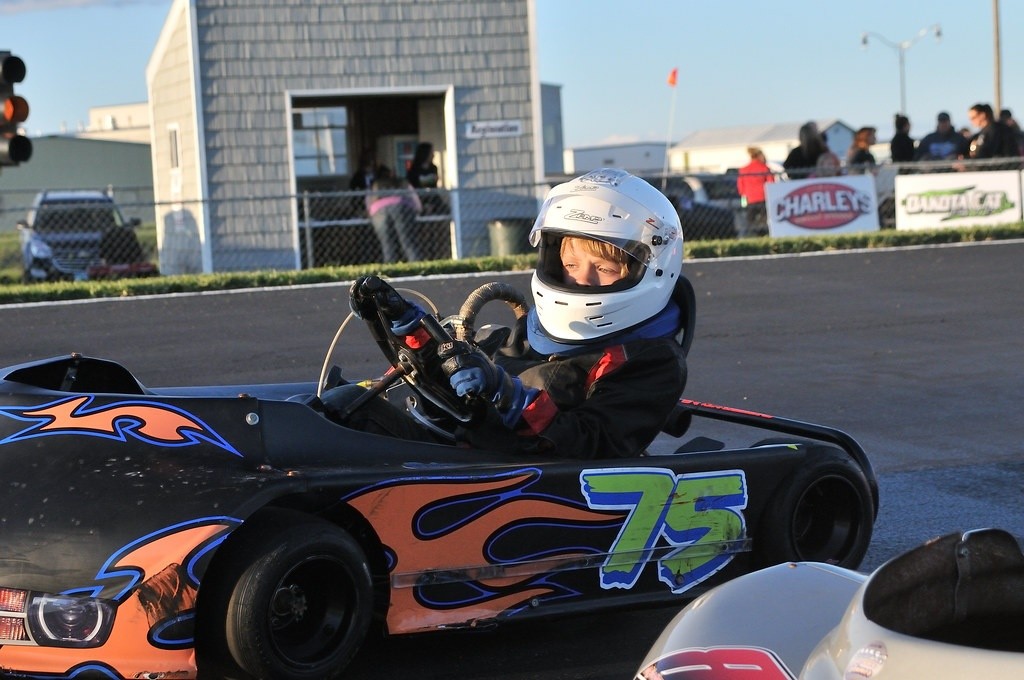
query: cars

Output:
[654,174,765,239]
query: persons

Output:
[959,128,971,140]
[918,111,967,162]
[737,147,775,240]
[890,112,918,164]
[998,109,1024,155]
[846,127,877,174]
[349,169,690,461]
[953,103,1003,170]
[783,121,841,180]
[352,138,443,263]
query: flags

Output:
[668,67,679,86]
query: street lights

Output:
[861,23,944,117]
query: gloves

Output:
[436,339,539,431]
[349,277,425,335]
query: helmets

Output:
[529,168,684,344]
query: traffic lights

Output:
[0,50,33,167]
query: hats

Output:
[938,112,950,121]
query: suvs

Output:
[16,187,142,283]
